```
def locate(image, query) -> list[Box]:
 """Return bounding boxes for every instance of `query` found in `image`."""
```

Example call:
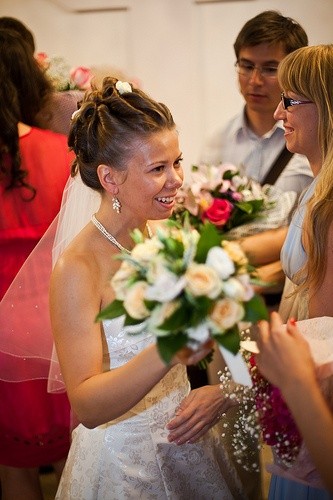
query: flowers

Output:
[114,79,132,96]
[216,327,303,476]
[91,210,278,370]
[170,152,295,236]
[36,49,92,92]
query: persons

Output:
[167,12,333,500]
[0,77,246,500]
[0,18,80,500]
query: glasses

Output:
[281,91,315,110]
[234,58,279,78]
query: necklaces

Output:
[91,214,154,255]
